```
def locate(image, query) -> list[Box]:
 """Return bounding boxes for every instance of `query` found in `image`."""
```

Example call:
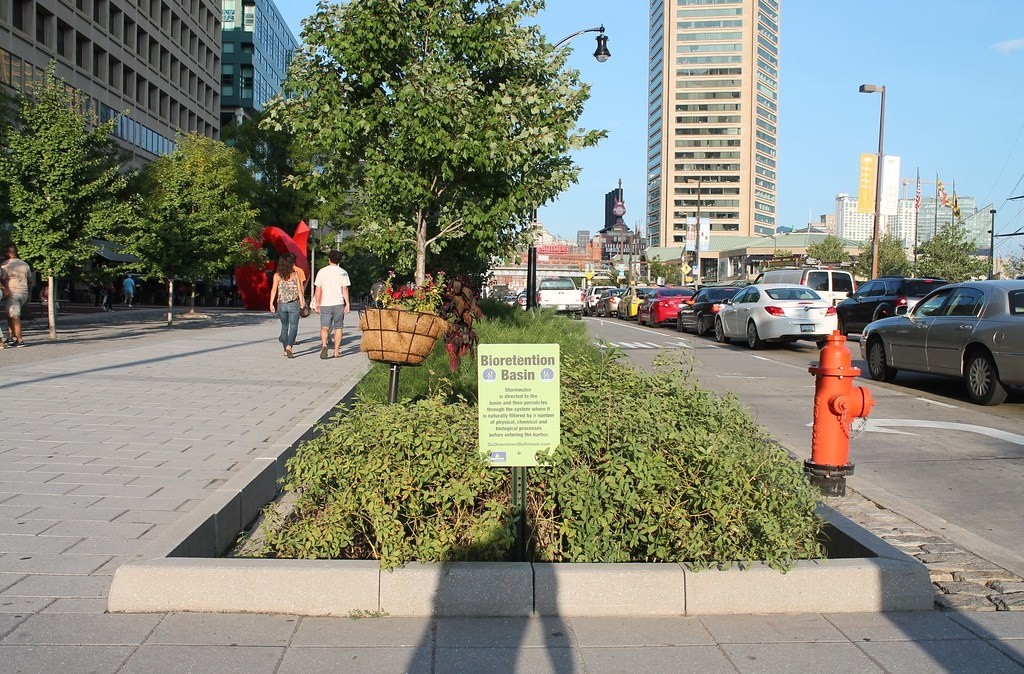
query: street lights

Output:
[335,235,342,265]
[525,23,611,313]
[695,178,700,283]
[771,235,776,252]
[859,83,885,280]
[990,210,997,279]
[309,219,318,301]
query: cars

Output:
[859,279,1024,406]
[595,286,654,320]
[714,283,838,350]
[676,285,744,337]
[637,288,693,328]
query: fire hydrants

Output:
[803,330,876,498]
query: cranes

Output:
[898,177,953,200]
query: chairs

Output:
[800,294,811,298]
[771,294,778,298]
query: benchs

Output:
[0,304,58,330]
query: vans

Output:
[748,266,857,309]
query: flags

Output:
[937,177,954,207]
[915,174,922,213]
[952,182,961,219]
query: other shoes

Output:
[4,338,14,345]
[109,310,115,312]
[320,346,328,359]
[284,351,288,356]
[7,340,25,348]
[0,342,7,349]
[285,348,293,357]
[128,304,133,308]
[293,341,300,345]
[333,352,340,358]
[101,305,107,312]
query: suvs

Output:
[583,285,617,316]
[835,274,952,336]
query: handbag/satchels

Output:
[300,304,310,317]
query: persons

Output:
[123,274,136,308]
[270,254,306,358]
[178,280,187,304]
[0,245,32,349]
[327,324,334,345]
[314,251,351,359]
[101,281,116,312]
[39,285,60,309]
[370,278,385,298]
[279,254,306,344]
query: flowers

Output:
[371,270,447,314]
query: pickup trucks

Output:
[536,278,586,320]
